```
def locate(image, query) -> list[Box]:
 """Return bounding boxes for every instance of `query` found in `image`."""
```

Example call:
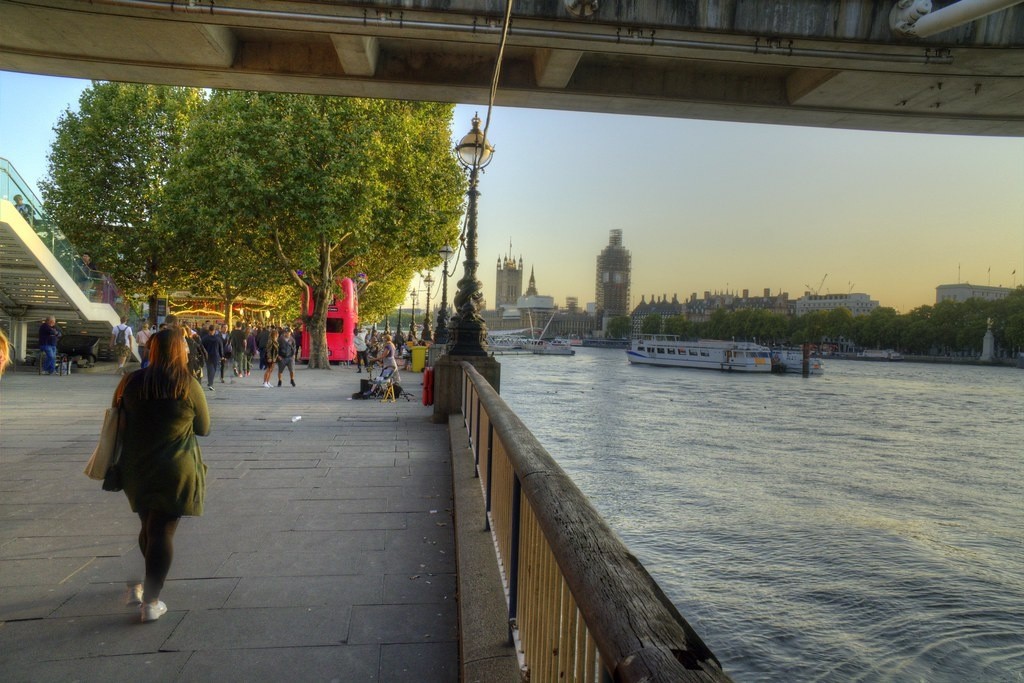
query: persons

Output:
[73,252,98,302]
[353,327,433,394]
[40,315,62,375]
[110,313,303,391]
[14,195,35,228]
[101,327,212,622]
[0,329,13,380]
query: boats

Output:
[494,338,576,356]
[770,347,825,376]
[625,332,772,371]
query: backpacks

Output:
[116,325,129,345]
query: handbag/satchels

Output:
[84,369,130,480]
[100,465,123,491]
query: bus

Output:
[301,276,358,362]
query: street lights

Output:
[422,271,434,341]
[408,287,418,341]
[433,238,454,343]
[451,111,496,353]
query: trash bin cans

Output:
[409,346,428,373]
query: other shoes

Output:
[221,377,225,383]
[290,379,296,386]
[277,380,283,387]
[357,369,361,373]
[263,382,273,388]
[234,367,242,378]
[207,384,214,390]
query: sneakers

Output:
[126,587,168,621]
